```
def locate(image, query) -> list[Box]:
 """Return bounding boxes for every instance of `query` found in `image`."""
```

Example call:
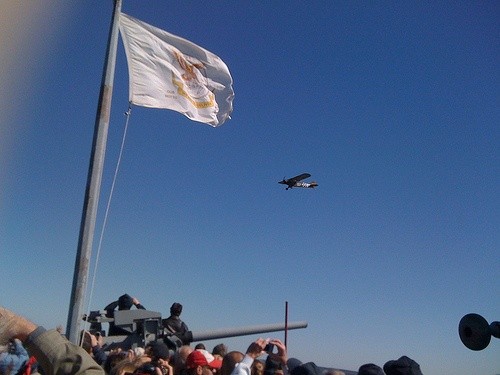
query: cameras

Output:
[263,344,274,353]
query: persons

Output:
[0,308,422,375]
[103,294,146,336]
[161,303,188,332]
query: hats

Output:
[186,349,224,368]
[383,356,422,375]
[287,358,320,375]
[359,363,383,375]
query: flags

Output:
[119,12,233,126]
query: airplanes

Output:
[277,172,319,191]
